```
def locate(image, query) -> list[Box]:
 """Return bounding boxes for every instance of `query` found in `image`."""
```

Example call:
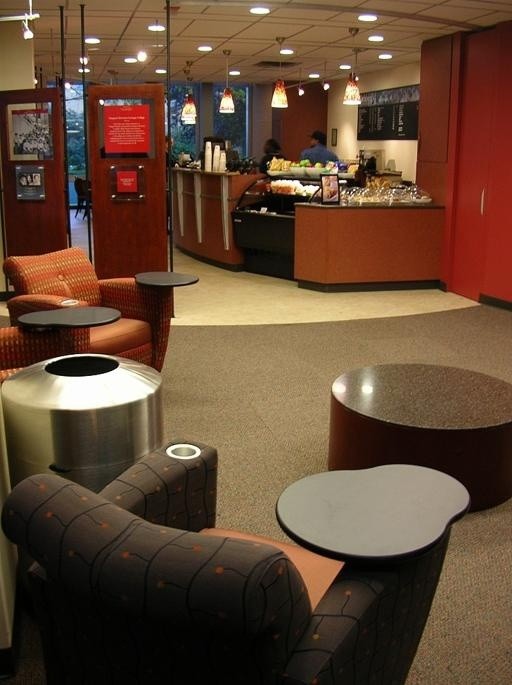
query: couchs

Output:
[1,439,470,685]
[6,247,200,377]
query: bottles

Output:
[13,132,23,154]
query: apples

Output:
[300,159,322,168]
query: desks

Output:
[292,198,446,290]
[130,272,201,377]
[20,300,121,350]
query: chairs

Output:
[70,176,87,221]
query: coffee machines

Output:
[356,149,377,187]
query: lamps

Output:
[0,0,44,44]
[272,35,290,110]
[219,53,238,116]
[342,26,364,108]
[183,84,198,128]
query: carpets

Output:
[155,301,510,684]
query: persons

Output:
[164,136,178,232]
[258,138,286,173]
[300,130,340,167]
[14,125,53,187]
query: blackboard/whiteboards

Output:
[357,84,419,140]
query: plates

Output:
[346,196,433,205]
[267,170,292,176]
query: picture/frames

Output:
[109,164,149,202]
[6,101,53,165]
[97,98,157,160]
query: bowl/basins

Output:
[305,168,330,178]
[290,166,306,177]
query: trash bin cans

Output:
[1,353,163,494]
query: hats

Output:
[308,131,327,141]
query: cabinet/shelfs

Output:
[231,176,333,278]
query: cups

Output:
[205,142,227,171]
[240,166,260,176]
